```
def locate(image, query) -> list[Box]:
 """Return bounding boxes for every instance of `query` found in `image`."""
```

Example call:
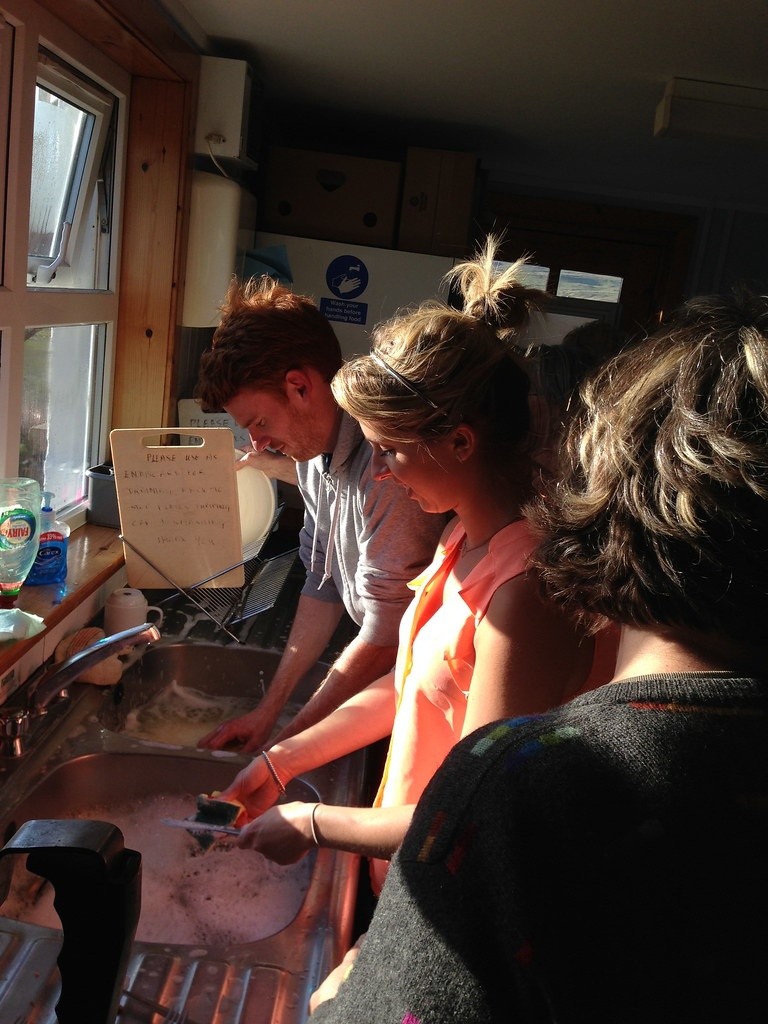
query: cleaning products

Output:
[0,477,42,608]
[22,492,70,586]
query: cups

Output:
[103,588,163,634]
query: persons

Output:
[202,266,602,903]
[196,276,448,756]
[306,316,768,1023]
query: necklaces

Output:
[460,534,492,558]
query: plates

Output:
[235,447,275,546]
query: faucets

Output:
[24,621,161,720]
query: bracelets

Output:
[261,751,286,793]
[310,802,323,850]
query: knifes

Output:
[160,816,241,836]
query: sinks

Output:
[57,642,332,751]
[1,752,358,962]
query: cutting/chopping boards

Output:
[109,426,245,589]
